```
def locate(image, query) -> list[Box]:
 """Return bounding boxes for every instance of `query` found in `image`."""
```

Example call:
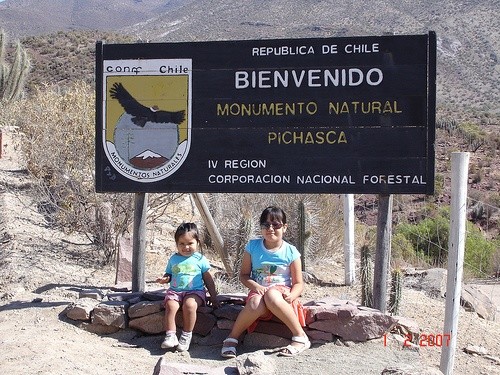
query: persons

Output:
[220,205,311,359]
[155,222,218,352]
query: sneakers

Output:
[177,331,193,353]
[161,333,180,349]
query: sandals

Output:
[220,338,240,358]
[279,337,312,356]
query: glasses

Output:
[260,222,285,230]
